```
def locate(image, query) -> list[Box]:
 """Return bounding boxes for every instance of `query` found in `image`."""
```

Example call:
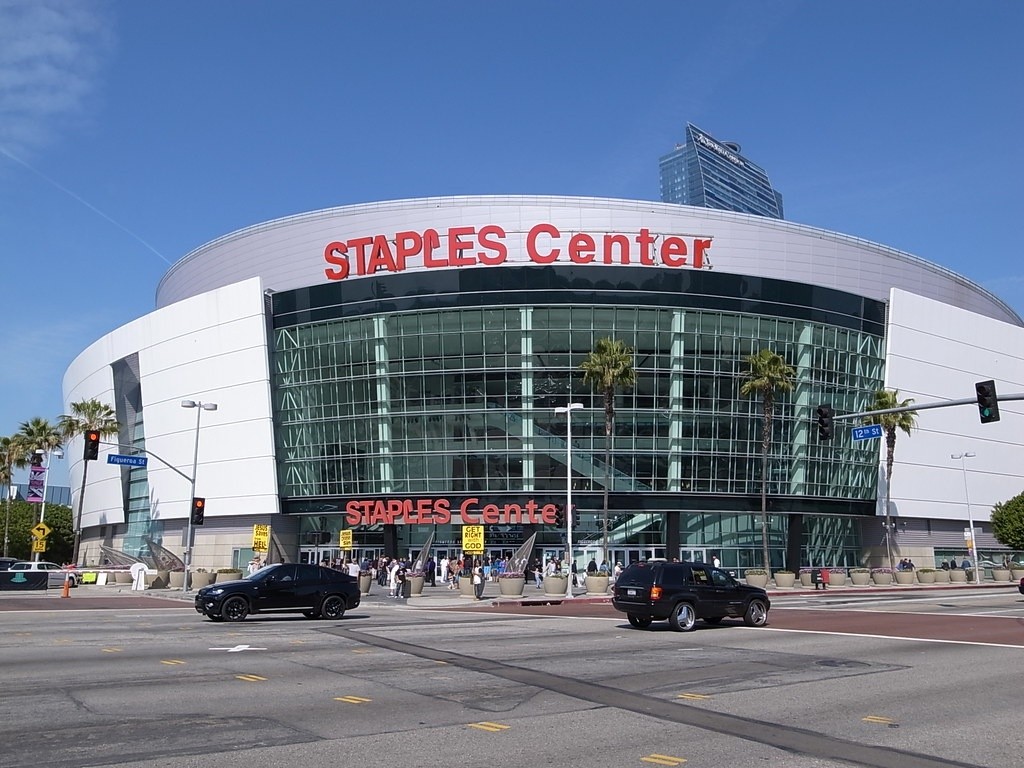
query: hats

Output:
[617,562,622,565]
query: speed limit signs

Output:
[32,540,46,552]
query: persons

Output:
[320,556,562,600]
[572,560,578,587]
[673,558,679,562]
[941,557,971,570]
[587,558,624,576]
[712,556,720,567]
[247,557,285,574]
[897,558,915,570]
[280,570,291,580]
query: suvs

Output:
[611,560,771,632]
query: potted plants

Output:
[774,571,795,587]
[916,568,967,586]
[991,564,1024,584]
[544,573,568,597]
[895,568,914,587]
[586,571,609,597]
[849,568,871,588]
[744,570,767,588]
[459,573,474,595]
[86,566,243,589]
[829,573,845,588]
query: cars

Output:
[195,563,360,622]
[1019,578,1024,594]
[8,561,77,588]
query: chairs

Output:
[693,574,704,586]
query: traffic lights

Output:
[190,497,205,526]
[84,430,101,460]
[975,380,1000,424]
[816,403,834,440]
[555,504,567,528]
[572,504,580,527]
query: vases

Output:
[359,575,371,593]
[800,574,813,586]
[406,577,425,594]
[873,573,892,587]
[499,577,525,595]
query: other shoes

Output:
[610,588,615,593]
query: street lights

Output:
[34,447,63,569]
[554,402,583,598]
[952,451,980,583]
[182,400,217,591]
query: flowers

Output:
[871,568,893,572]
[500,571,525,578]
[829,569,844,574]
[405,571,426,578]
[359,569,372,577]
[799,569,816,574]
